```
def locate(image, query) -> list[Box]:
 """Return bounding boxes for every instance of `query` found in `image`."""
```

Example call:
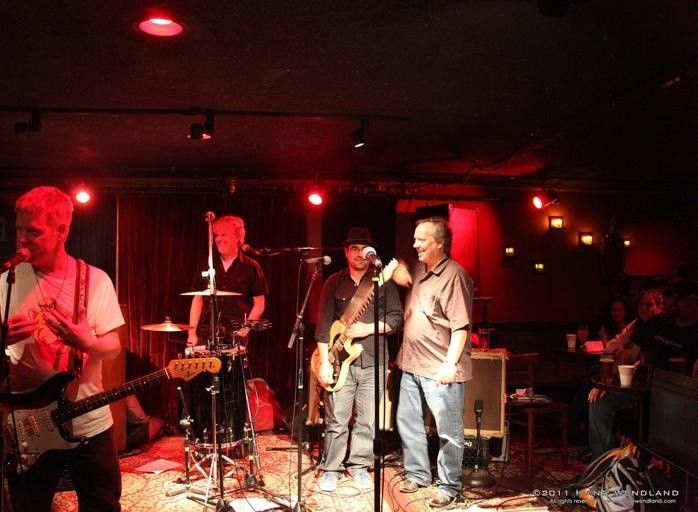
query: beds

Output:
[587,362,656,452]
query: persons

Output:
[392,216,471,508]
[598,296,628,341]
[185,215,269,349]
[587,282,698,460]
[315,225,404,493]
[0,185,126,512]
[636,288,664,321]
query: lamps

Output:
[15,108,42,137]
[548,216,566,234]
[352,117,371,150]
[575,233,599,254]
[132,3,184,36]
[529,192,562,215]
[502,242,519,265]
[188,112,214,141]
[528,255,547,276]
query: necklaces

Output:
[30,255,70,308]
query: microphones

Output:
[242,245,262,257]
[362,246,382,271]
[300,256,332,265]
[474,399,485,416]
[2,248,32,272]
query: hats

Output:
[338,227,375,246]
[662,280,697,297]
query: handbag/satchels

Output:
[560,445,655,511]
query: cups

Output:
[618,365,634,385]
[566,334,577,348]
[478,332,490,351]
[577,327,589,345]
[600,359,615,379]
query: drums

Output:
[185,345,248,449]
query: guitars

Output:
[0,353,222,481]
[311,258,399,393]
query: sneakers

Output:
[354,470,375,493]
[399,481,421,492]
[429,491,454,508]
[319,474,340,490]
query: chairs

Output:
[501,352,569,479]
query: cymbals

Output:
[141,322,195,332]
[181,289,243,297]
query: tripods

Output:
[208,338,296,500]
[187,222,235,512]
[166,386,215,497]
[294,388,326,480]
[177,298,247,512]
[289,264,323,512]
[260,247,319,472]
